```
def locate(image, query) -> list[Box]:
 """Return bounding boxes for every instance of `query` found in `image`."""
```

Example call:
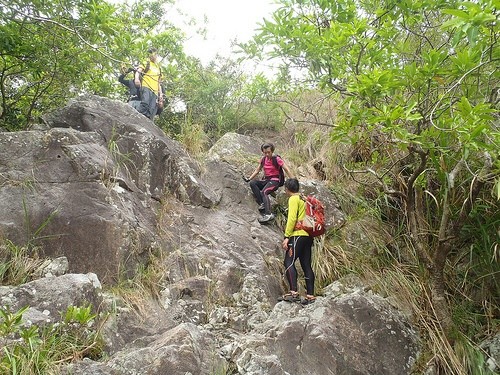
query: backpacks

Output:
[294,195,325,236]
[261,154,285,186]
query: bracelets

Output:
[284,237,290,240]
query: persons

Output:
[119,49,166,122]
[276,178,316,304]
[243,142,294,225]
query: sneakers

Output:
[276,293,304,303]
[257,214,274,227]
[302,298,318,306]
[258,203,265,210]
